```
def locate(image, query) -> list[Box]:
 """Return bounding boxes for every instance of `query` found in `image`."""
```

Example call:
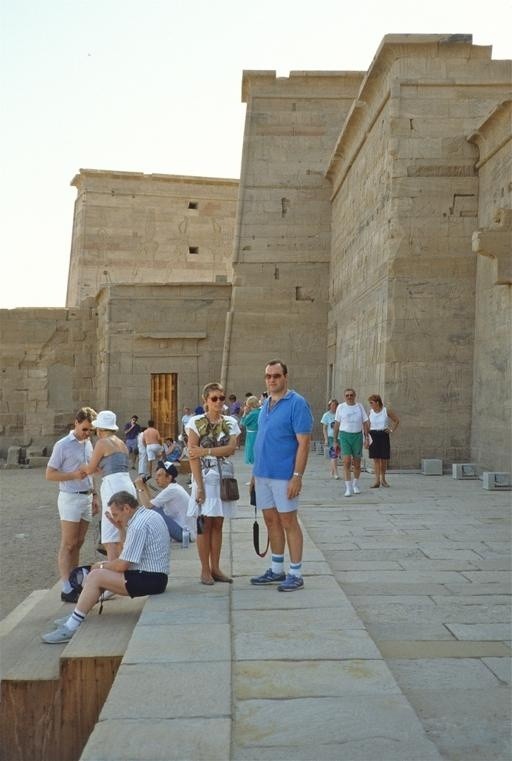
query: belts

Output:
[79,492,91,494]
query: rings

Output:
[190,451,191,453]
[199,498,201,500]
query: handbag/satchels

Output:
[220,478,239,501]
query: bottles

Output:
[182,525,189,548]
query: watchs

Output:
[138,488,144,492]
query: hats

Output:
[69,566,92,593]
[92,411,119,431]
[158,460,178,477]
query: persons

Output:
[185,383,242,584]
[321,399,340,479]
[81,411,137,600]
[363,394,398,488]
[334,388,369,497]
[45,407,100,604]
[134,461,198,542]
[182,406,206,435]
[123,416,188,479]
[41,491,170,643]
[223,391,268,484]
[250,361,312,592]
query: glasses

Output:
[211,396,226,402]
[264,373,281,380]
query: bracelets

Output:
[293,473,303,477]
[209,448,211,455]
[365,436,368,439]
[390,430,393,432]
[93,493,98,495]
[100,562,104,569]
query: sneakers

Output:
[353,486,360,493]
[215,574,233,583]
[54,615,72,626]
[344,490,351,496]
[42,625,74,644]
[250,568,286,584]
[278,574,304,591]
[201,575,215,584]
[61,589,79,603]
